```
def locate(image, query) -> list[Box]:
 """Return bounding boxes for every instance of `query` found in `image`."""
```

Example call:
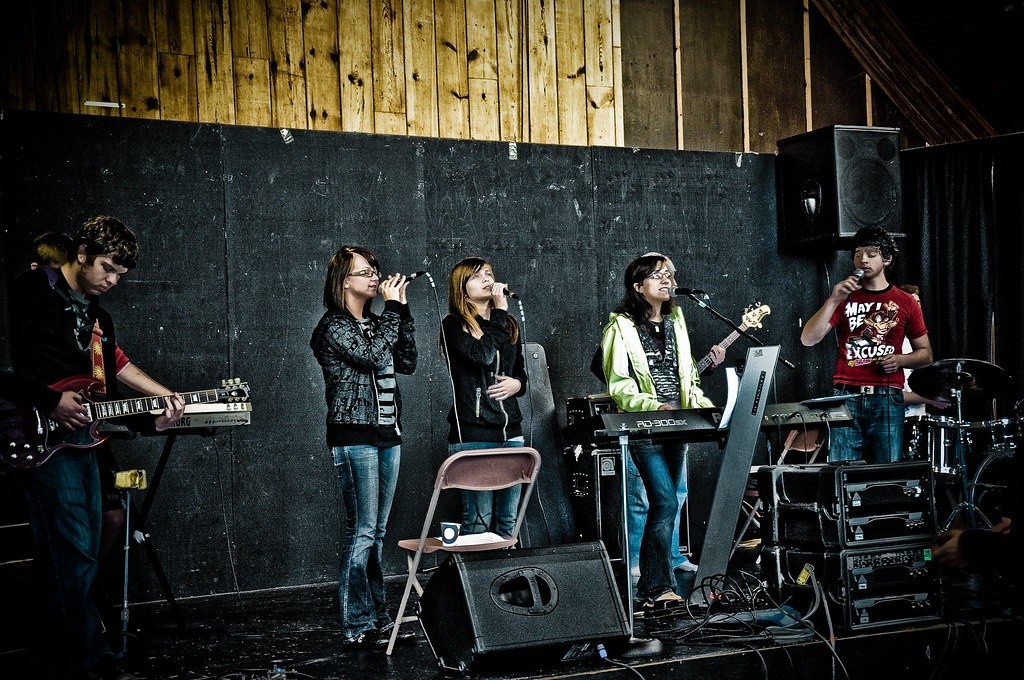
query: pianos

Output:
[93,401,253,637]
[561,392,862,646]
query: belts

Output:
[833,382,902,395]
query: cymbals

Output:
[906,358,1016,406]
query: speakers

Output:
[565,446,692,561]
[414,540,631,679]
[776,124,907,246]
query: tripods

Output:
[936,381,994,537]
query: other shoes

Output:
[382,625,416,639]
[656,590,682,602]
[98,662,130,680]
[353,631,388,645]
[679,561,699,573]
[631,566,641,577]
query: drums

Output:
[977,397,1024,458]
[968,451,1024,532]
[901,414,972,485]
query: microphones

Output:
[845,269,865,302]
[668,286,705,297]
[489,283,520,301]
[378,271,427,294]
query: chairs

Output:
[727,426,827,565]
[385,447,542,656]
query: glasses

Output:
[347,269,383,278]
[646,271,672,280]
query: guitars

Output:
[696,301,772,375]
[0,377,251,471]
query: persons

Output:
[932,464,1024,597]
[895,284,951,422]
[799,223,934,463]
[0,215,184,676]
[439,258,529,551]
[590,250,726,616]
[309,245,417,648]
